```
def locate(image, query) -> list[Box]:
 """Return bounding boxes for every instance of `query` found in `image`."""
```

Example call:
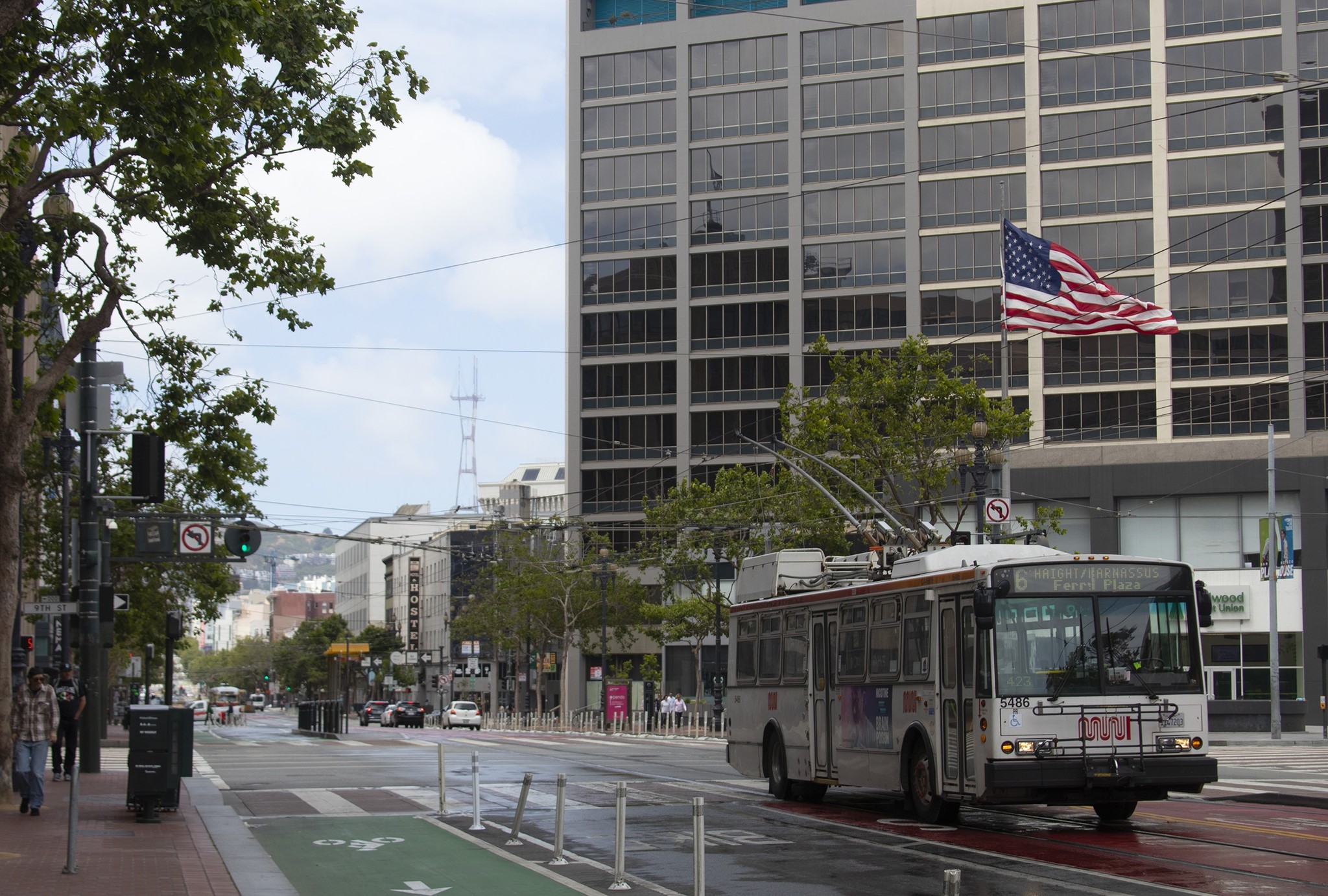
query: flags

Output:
[1000,218,1178,335]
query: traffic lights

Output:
[20,635,36,649]
[287,682,290,691]
[224,520,262,559]
[264,670,269,680]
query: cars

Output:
[379,704,395,726]
[141,684,265,722]
[426,710,440,723]
[442,701,482,731]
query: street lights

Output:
[442,602,463,701]
[690,523,738,733]
[954,409,1005,543]
[590,545,617,729]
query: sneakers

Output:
[53,773,61,781]
[64,774,71,780]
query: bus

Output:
[722,430,1219,828]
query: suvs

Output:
[359,700,390,726]
[389,700,425,728]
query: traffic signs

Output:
[419,651,433,666]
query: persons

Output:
[9,666,60,816]
[661,695,671,729]
[673,693,687,729]
[1104,639,1142,672]
[654,693,663,729]
[225,700,233,724]
[1281,529,1288,578]
[1262,537,1270,580]
[204,702,214,726]
[668,692,675,727]
[51,662,86,782]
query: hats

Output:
[28,666,45,679]
[60,662,72,671]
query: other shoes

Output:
[30,808,40,815]
[20,798,29,813]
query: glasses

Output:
[33,677,45,682]
